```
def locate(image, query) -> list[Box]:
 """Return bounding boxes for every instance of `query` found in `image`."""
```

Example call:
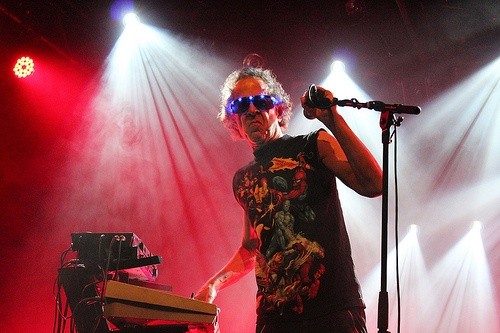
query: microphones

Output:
[303,86,318,119]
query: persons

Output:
[192,66,387,333]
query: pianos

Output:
[58,267,220,333]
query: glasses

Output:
[228,94,280,114]
